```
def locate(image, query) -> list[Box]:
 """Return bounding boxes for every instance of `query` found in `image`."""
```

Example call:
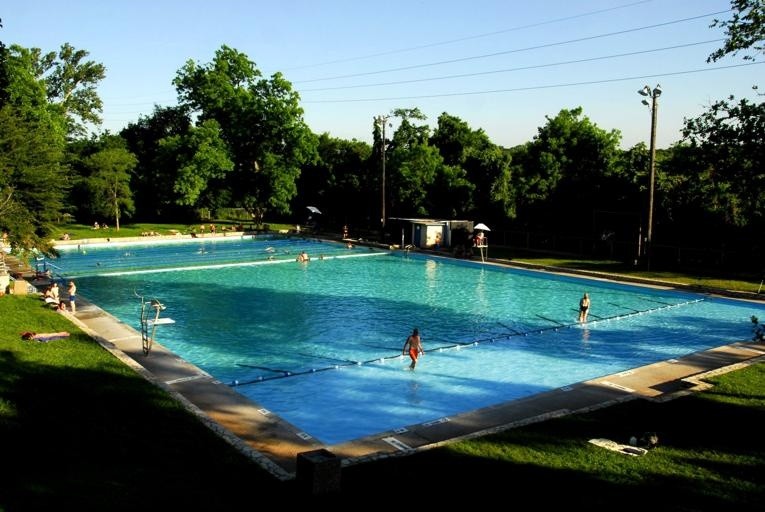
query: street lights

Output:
[370,110,392,243]
[636,79,664,272]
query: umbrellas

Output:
[474,222,491,232]
[305,205,323,223]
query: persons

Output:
[50,282,59,300]
[90,221,100,231]
[141,222,265,238]
[343,225,348,239]
[301,252,309,263]
[402,328,425,370]
[435,235,442,255]
[472,230,485,247]
[347,241,352,249]
[43,286,60,311]
[58,300,67,311]
[102,224,109,229]
[68,280,77,312]
[296,253,304,263]
[578,292,591,322]
